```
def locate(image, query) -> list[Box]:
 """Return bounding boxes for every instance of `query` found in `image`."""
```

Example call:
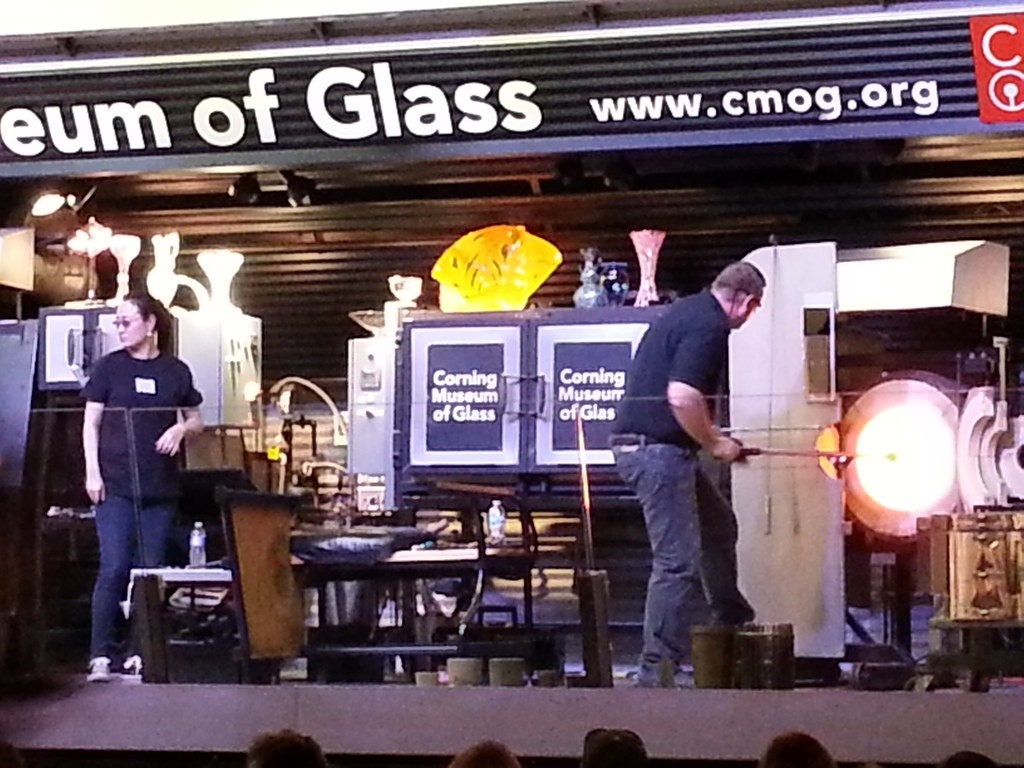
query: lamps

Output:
[278,170,320,209]
[20,176,67,218]
[225,173,264,203]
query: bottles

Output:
[190,522,206,568]
[488,500,506,541]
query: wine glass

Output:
[105,234,143,307]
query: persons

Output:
[760,731,837,768]
[608,260,766,687]
[77,290,204,684]
[244,728,330,768]
[449,737,523,768]
[580,729,649,768]
[939,749,1002,768]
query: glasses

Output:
[112,315,137,328]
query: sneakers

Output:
[82,657,114,683]
[119,656,143,681]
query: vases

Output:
[629,229,665,306]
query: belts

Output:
[609,435,670,447]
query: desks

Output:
[124,544,588,684]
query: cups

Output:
[447,657,483,687]
[389,276,422,305]
[489,658,525,688]
[631,229,665,308]
[197,249,245,309]
[690,622,737,689]
[415,672,439,686]
[733,621,795,688]
